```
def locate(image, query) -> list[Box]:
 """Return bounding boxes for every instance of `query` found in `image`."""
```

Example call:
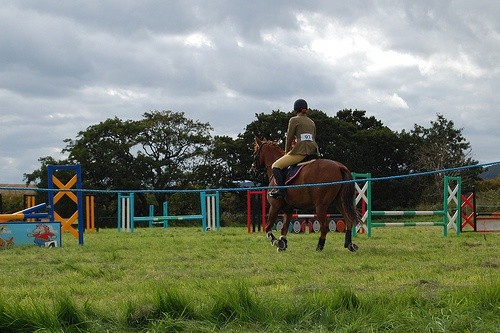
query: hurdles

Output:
[117,191,219,231]
[458,188,499,232]
[351,171,460,237]
[23,193,98,232]
[1,163,83,245]
[247,190,365,235]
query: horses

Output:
[251,132,361,255]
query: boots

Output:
[271,167,286,197]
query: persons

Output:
[270,98,318,197]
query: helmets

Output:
[294,99,307,111]
